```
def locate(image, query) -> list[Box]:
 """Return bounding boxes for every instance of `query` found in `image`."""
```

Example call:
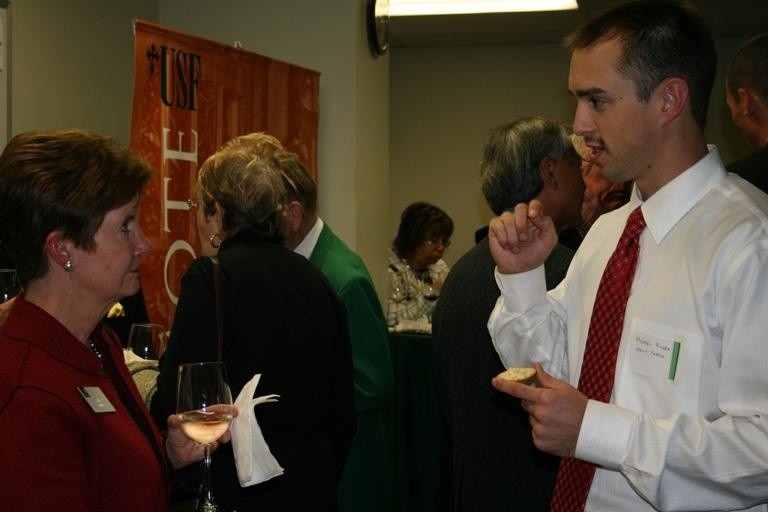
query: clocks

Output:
[368,0,391,54]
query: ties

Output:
[549,208,647,512]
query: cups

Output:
[128,322,167,360]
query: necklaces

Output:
[89,340,103,367]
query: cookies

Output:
[497,367,536,382]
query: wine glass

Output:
[174,360,234,512]
[385,263,440,327]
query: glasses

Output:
[426,238,452,249]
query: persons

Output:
[279,2,768,512]
[146,132,351,512]
[0,132,239,512]
